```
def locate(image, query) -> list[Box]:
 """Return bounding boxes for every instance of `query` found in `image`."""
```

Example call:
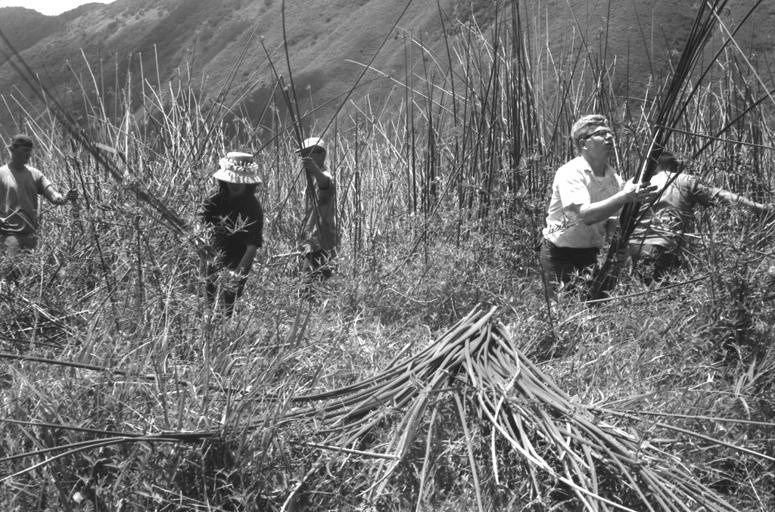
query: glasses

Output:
[584,129,615,139]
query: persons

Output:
[294,137,338,303]
[193,151,264,318]
[539,114,658,300]
[0,134,79,257]
[628,152,775,287]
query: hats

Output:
[294,136,328,155]
[212,151,263,187]
[5,134,34,149]
